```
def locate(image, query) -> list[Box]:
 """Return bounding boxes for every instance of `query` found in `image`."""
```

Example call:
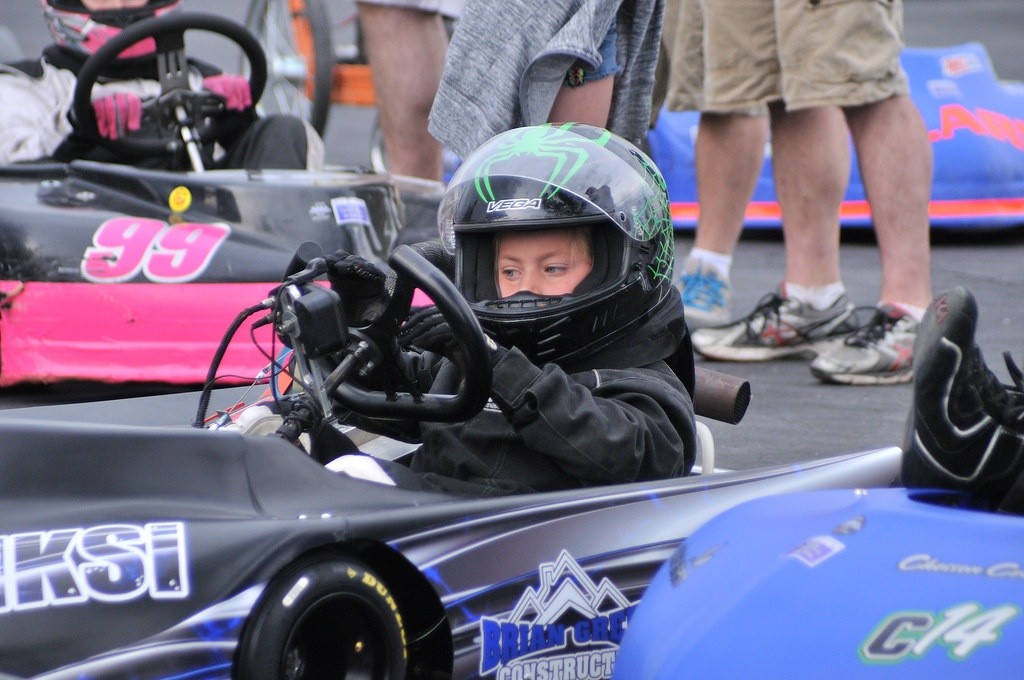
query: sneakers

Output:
[900,286,1024,516]
[690,283,865,362]
[677,258,732,326]
[809,301,965,386]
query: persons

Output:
[323,121,697,484]
[2,2,310,171]
[899,284,1024,504]
[356,0,932,387]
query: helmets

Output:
[436,121,686,375]
[38,0,183,84]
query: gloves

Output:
[396,304,510,378]
[67,92,143,141]
[323,246,391,326]
[203,74,253,112]
